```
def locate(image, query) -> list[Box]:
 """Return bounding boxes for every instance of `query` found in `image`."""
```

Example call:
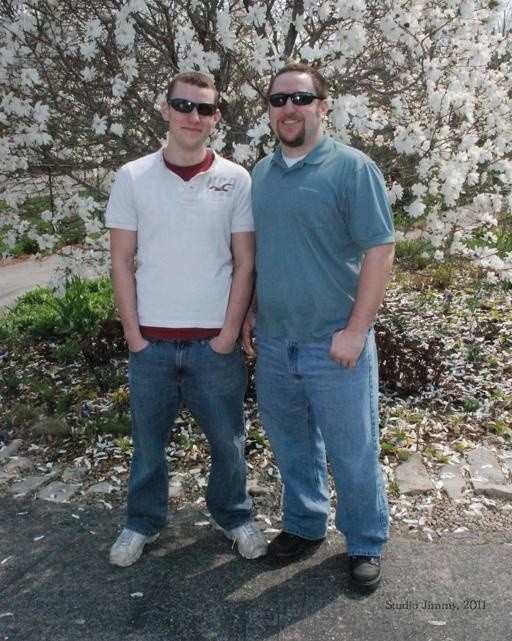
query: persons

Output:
[238,66,396,593]
[104,71,257,568]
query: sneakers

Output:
[108,529,160,567]
[224,521,267,559]
[348,555,382,589]
[268,531,327,556]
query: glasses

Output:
[165,99,217,117]
[268,93,322,107]
